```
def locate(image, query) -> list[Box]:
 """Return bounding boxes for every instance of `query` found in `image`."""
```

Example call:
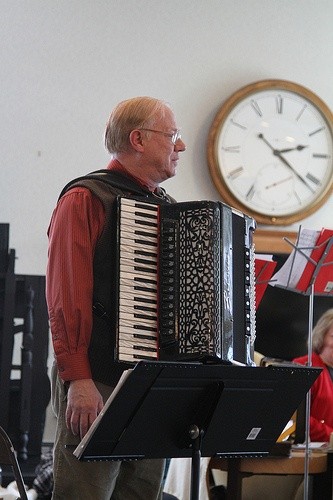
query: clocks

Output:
[207,79,332,226]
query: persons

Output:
[289,307,333,443]
[45,97,188,500]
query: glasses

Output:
[133,127,182,145]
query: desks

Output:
[205,443,327,500]
